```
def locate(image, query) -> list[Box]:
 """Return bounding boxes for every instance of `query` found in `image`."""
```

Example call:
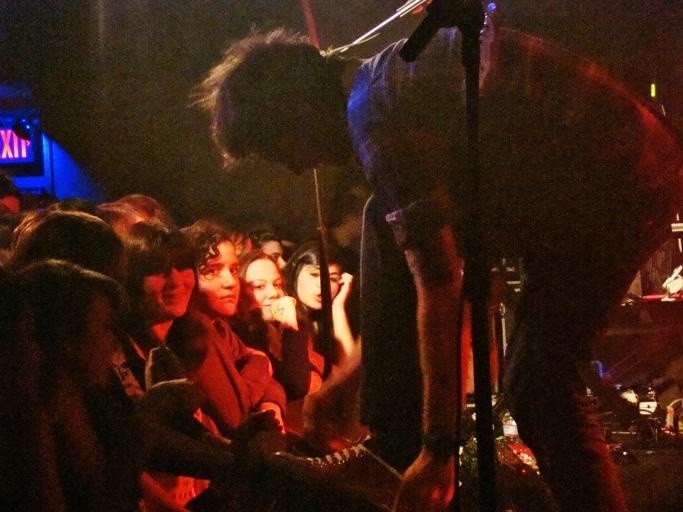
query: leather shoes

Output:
[269,443,405,512]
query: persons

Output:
[0,187,354,511]
[207,20,682,512]
